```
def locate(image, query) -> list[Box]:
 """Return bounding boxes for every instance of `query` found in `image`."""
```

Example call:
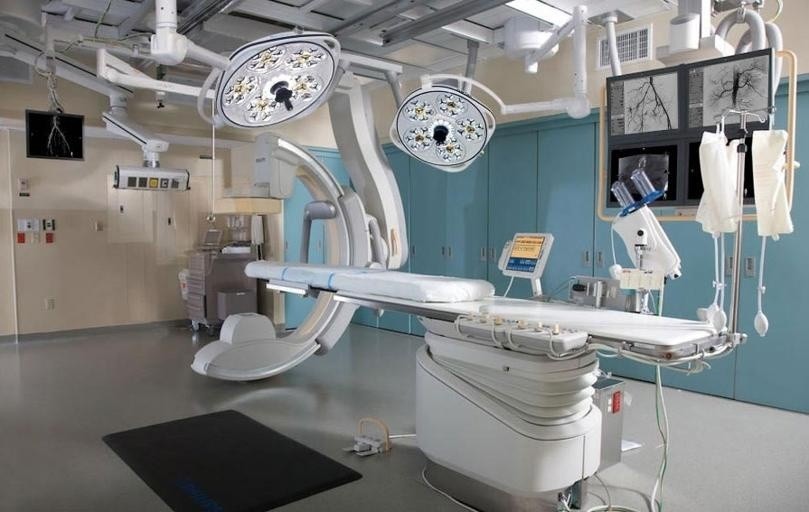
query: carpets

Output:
[103,410,363,512]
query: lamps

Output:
[389,4,591,173]
[150,0,341,130]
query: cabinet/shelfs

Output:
[183,243,257,336]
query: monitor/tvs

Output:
[24,109,85,161]
[502,232,554,280]
[603,48,776,218]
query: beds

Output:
[244,260,747,512]
